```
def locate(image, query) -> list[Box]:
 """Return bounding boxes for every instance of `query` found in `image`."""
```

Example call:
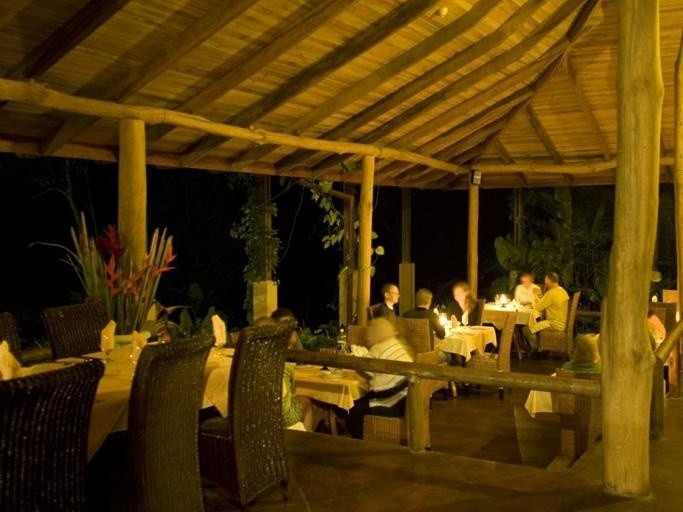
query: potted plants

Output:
[30,209,176,362]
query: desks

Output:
[292,364,372,436]
[1,342,235,465]
[433,325,498,389]
[481,302,543,360]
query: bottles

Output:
[336,324,347,353]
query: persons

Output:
[348,318,414,439]
[515,271,541,308]
[449,283,482,331]
[524,271,569,360]
[271,307,305,353]
[256,317,313,433]
[375,283,399,329]
[402,288,451,365]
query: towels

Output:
[100,319,117,352]
[0,339,21,381]
[461,310,469,325]
[211,314,227,346]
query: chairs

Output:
[398,317,457,399]
[363,350,436,447]
[463,312,512,400]
[198,320,292,512]
[87,331,215,512]
[537,290,581,361]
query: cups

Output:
[328,347,338,356]
[318,348,327,371]
